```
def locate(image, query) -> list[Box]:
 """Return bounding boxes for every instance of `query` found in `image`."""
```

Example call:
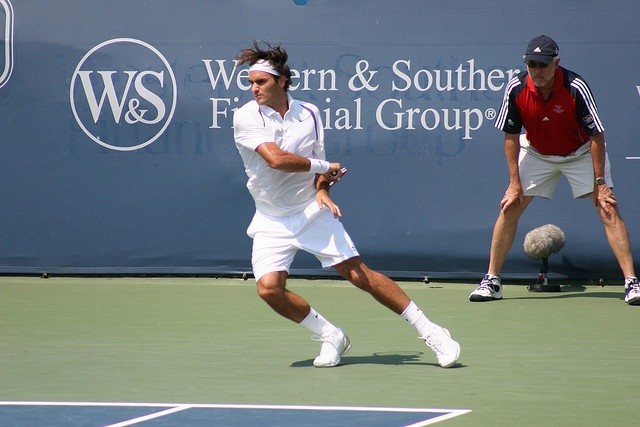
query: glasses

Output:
[525,61,551,70]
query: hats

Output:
[523,36,558,64]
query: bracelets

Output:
[308,157,330,174]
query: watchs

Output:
[593,177,605,184]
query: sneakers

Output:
[311,327,351,366]
[468,274,503,301]
[418,328,460,368]
[624,276,640,304]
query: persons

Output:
[233,40,460,366]
[469,34,640,304]
